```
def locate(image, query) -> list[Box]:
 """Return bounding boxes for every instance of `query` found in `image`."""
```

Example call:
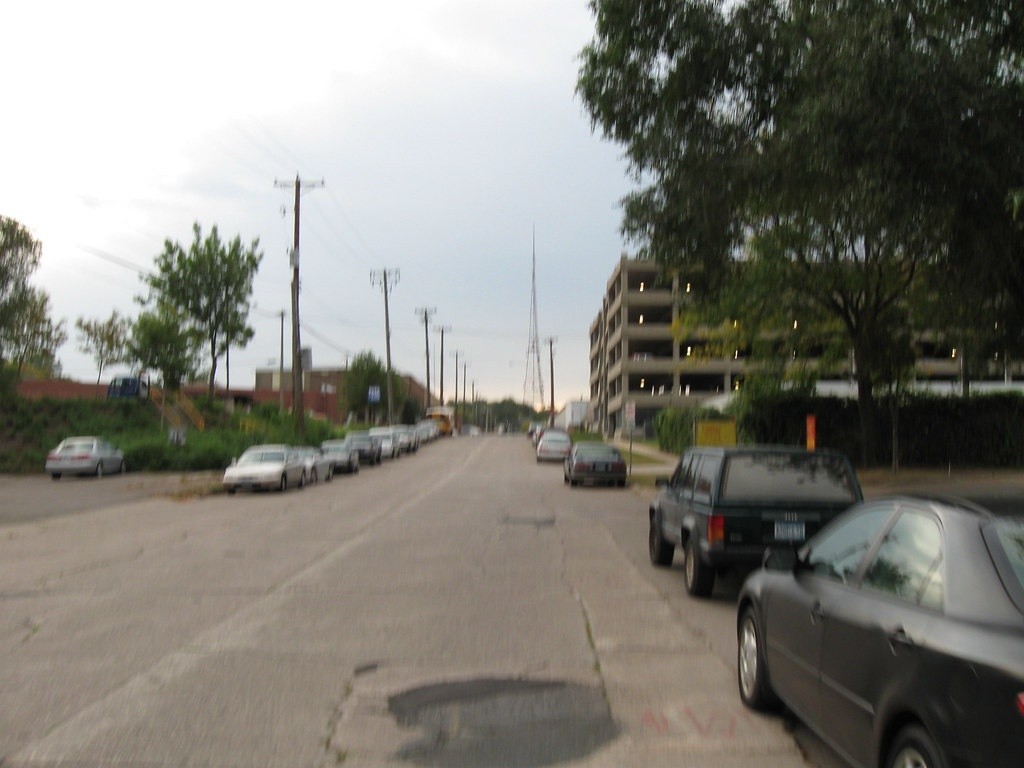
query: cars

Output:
[45,435,127,480]
[222,442,307,495]
[563,441,627,488]
[289,418,441,484]
[734,489,1024,768]
[536,429,572,463]
[527,421,547,448]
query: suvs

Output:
[646,444,866,598]
